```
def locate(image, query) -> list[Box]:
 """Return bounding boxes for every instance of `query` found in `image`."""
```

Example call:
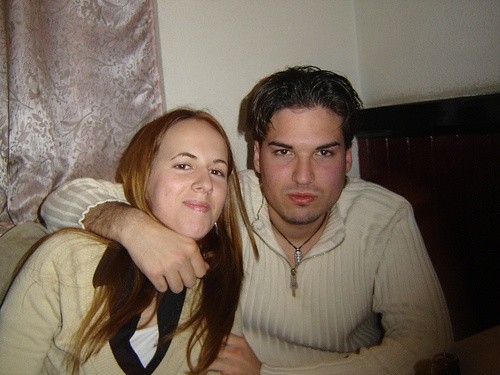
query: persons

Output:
[1,105,259,373]
[38,64,459,374]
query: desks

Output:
[452,324,500,375]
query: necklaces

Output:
[269,210,328,267]
